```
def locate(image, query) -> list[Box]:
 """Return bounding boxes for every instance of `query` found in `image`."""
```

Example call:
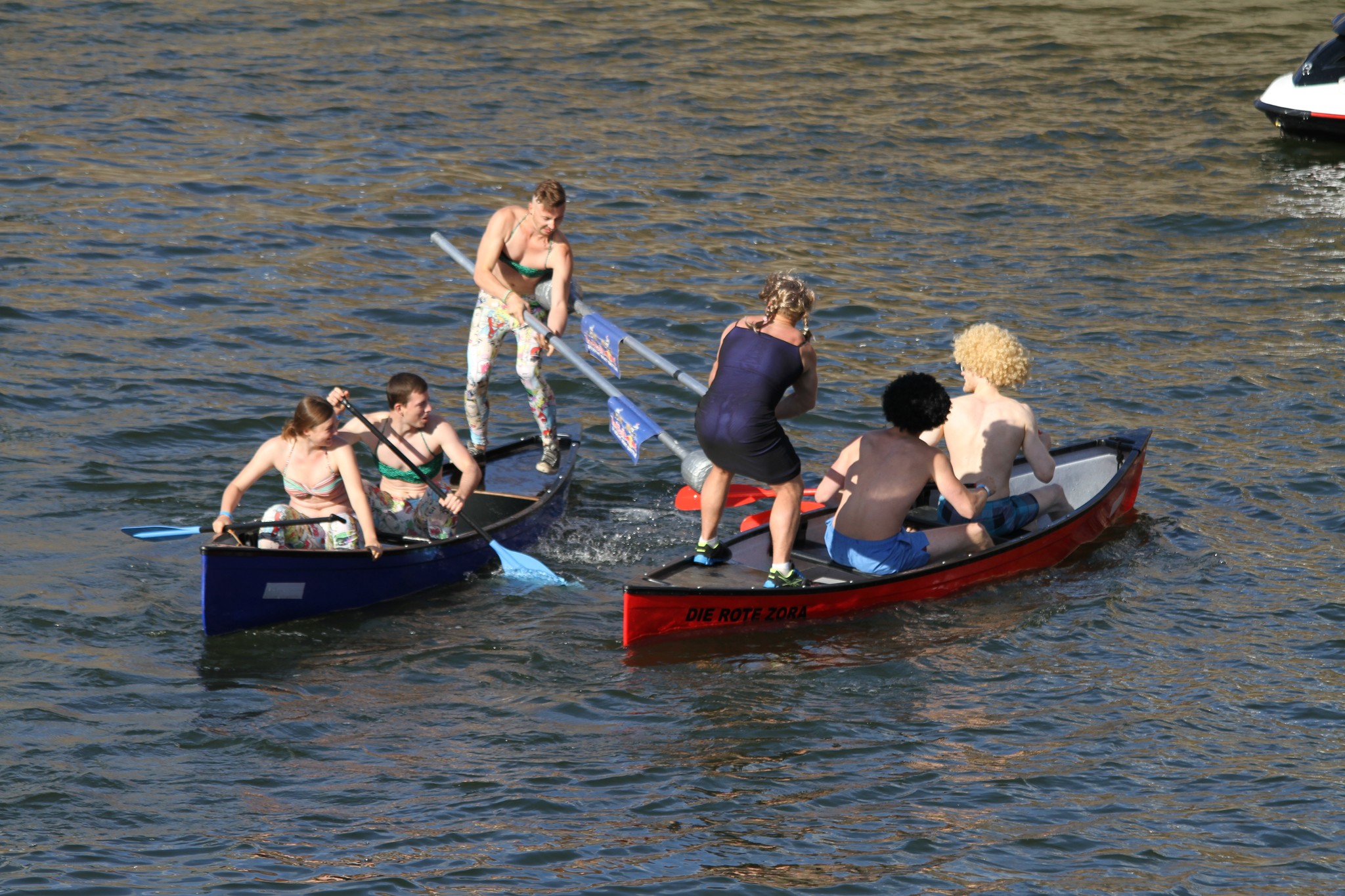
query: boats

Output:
[622,423,1156,646]
[199,430,583,639]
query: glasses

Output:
[959,364,968,371]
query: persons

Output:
[326,372,482,543]
[920,325,1076,539]
[694,269,819,590]
[210,396,382,561]
[813,371,998,575]
[466,180,574,473]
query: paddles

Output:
[675,483,977,511]
[740,430,1042,534]
[120,514,347,543]
[342,395,556,578]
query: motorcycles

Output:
[1254,15,1345,141]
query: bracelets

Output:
[975,485,991,497]
[503,291,513,304]
[219,511,233,521]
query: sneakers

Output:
[694,538,732,566]
[763,560,820,589]
[450,440,486,464]
[536,441,561,475]
